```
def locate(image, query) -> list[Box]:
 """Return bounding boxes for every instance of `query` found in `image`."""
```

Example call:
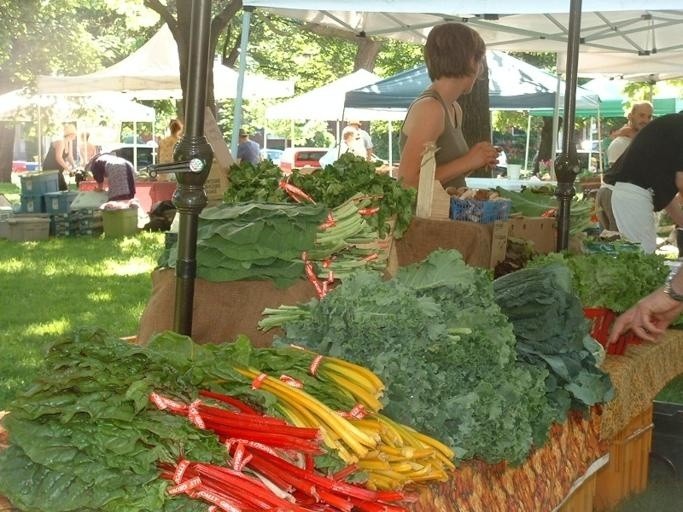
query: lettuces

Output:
[526,249,672,315]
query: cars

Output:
[579,136,604,151]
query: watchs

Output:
[663,266,683,302]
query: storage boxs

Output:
[0,160,138,241]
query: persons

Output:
[77,131,96,168]
[237,129,264,169]
[492,146,508,179]
[42,123,77,191]
[594,101,683,256]
[318,131,354,170]
[84,153,136,202]
[397,22,503,193]
[340,120,374,164]
[608,265,683,345]
[156,119,183,165]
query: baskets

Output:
[451,195,513,224]
[50,209,104,239]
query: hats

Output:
[63,119,362,136]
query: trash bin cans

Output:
[648,371,682,495]
[99,200,139,240]
